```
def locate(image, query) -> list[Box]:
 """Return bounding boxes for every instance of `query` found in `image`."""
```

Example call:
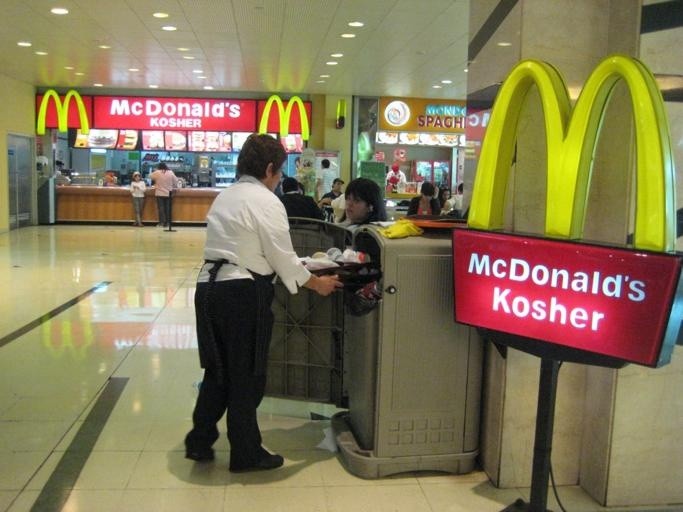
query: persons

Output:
[130,172,147,227]
[407,167,464,216]
[185,133,344,473]
[278,178,325,221]
[298,182,305,196]
[317,178,344,211]
[105,170,118,184]
[56,160,63,171]
[148,162,177,227]
[314,159,335,202]
[344,176,394,236]
[383,164,406,195]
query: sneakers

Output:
[133,222,169,227]
[181,434,217,462]
[226,454,285,473]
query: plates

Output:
[384,100,410,127]
[378,131,465,148]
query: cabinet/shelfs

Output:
[210,161,240,188]
[142,160,193,189]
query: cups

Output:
[311,247,343,263]
[416,207,422,217]
[425,207,433,217]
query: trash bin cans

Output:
[38,177,57,225]
[332,218,484,480]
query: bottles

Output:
[345,250,373,263]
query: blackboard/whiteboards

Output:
[361,161,385,199]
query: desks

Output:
[323,205,336,223]
[399,214,468,229]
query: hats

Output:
[132,171,141,179]
